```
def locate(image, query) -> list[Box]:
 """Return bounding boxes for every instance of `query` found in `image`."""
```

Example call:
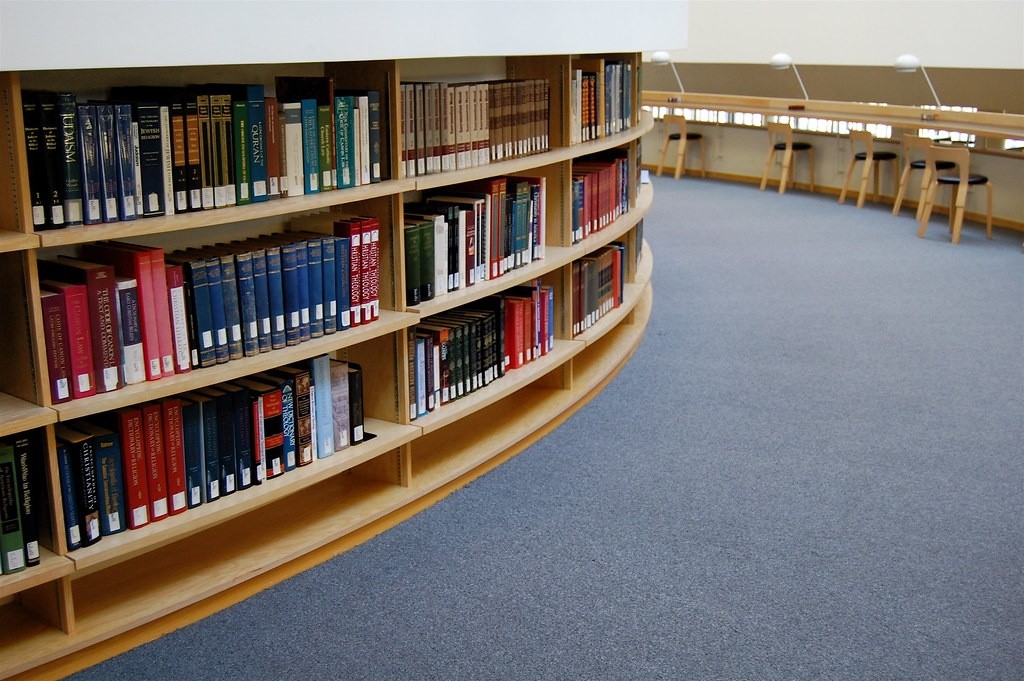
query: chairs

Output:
[892,134,959,221]
[839,131,899,209]
[918,145,993,243]
[760,123,814,195]
[656,115,706,180]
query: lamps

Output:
[894,56,940,109]
[652,51,684,93]
[770,53,809,101]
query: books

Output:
[0,60,647,578]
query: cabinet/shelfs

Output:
[0,53,655,681]
